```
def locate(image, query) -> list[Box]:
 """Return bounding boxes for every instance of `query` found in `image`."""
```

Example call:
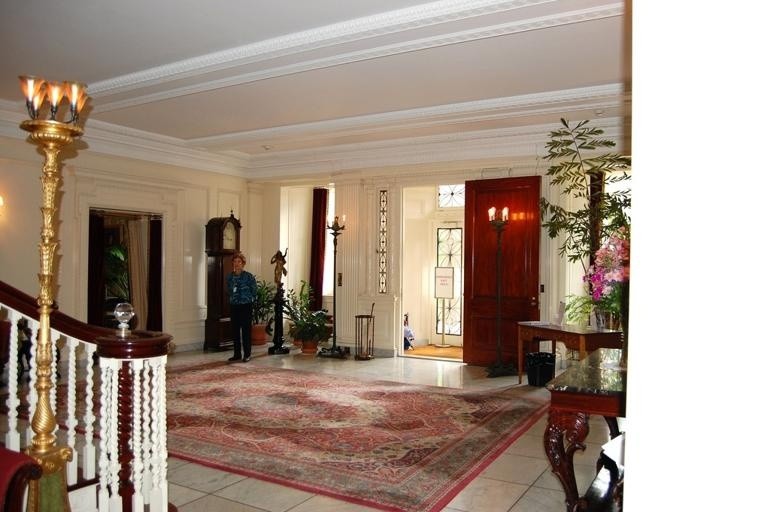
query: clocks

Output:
[203,208,243,353]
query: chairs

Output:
[0,445,43,512]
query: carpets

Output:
[0,361,551,512]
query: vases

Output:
[619,333,628,367]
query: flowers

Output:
[582,224,630,334]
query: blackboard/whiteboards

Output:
[435,267,454,299]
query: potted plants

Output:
[252,275,277,345]
[283,279,314,347]
[288,308,333,354]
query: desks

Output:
[518,321,624,384]
[544,347,628,512]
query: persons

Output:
[225,253,258,363]
[271,247,289,289]
[88,266,109,328]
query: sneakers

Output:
[228,355,242,361]
[243,356,251,362]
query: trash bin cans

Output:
[526,352,556,386]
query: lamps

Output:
[15,73,89,512]
[318,215,347,359]
[485,206,517,378]
